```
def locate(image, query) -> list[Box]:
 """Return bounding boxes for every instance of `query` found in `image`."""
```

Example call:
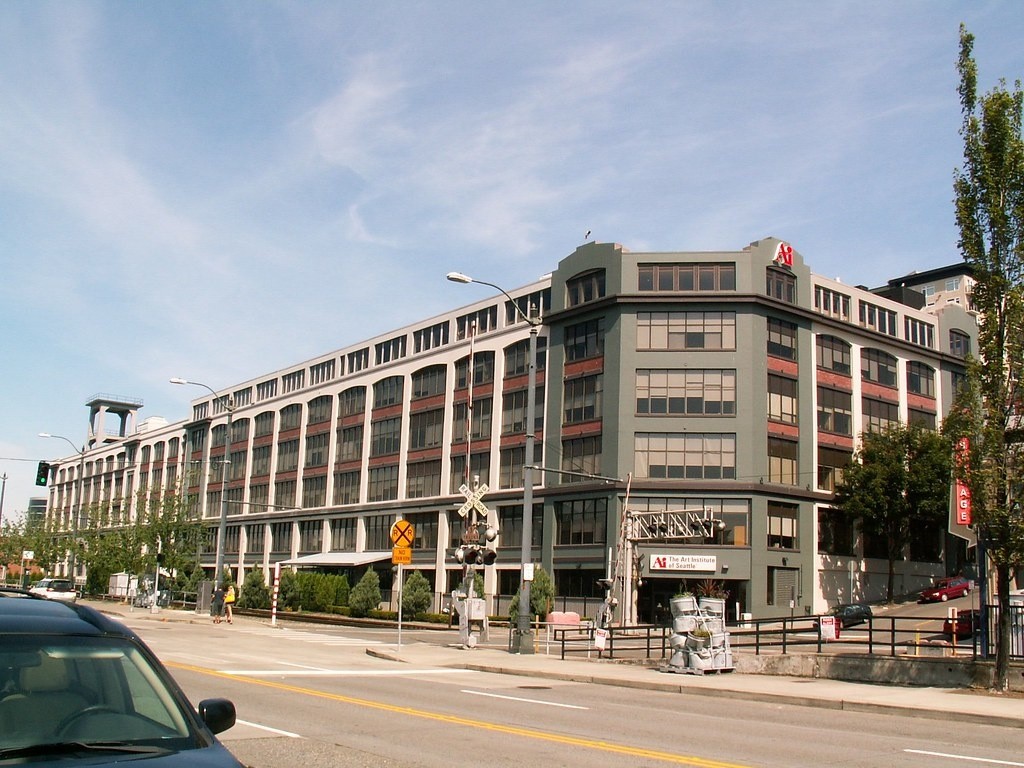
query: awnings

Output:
[278,552,392,567]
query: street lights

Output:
[39,432,85,593]
[445,271,552,652]
[169,376,235,616]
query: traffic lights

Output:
[611,603,618,609]
[463,547,497,566]
[36,462,49,487]
[601,613,609,624]
[604,581,613,590]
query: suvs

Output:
[28,577,77,603]
[0,586,252,768]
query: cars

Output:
[943,608,983,641]
[919,576,970,603]
[813,603,873,631]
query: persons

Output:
[210,586,235,624]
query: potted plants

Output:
[698,575,730,612]
[670,578,696,612]
